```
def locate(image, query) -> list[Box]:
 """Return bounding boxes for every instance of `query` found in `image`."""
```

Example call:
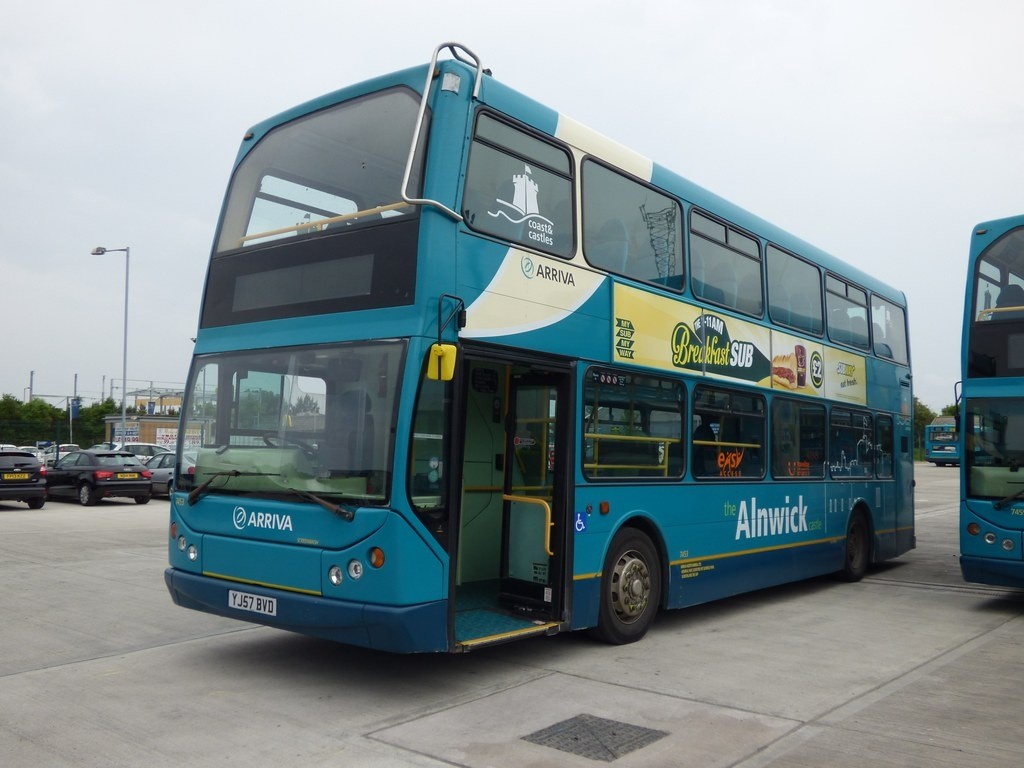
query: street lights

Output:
[91,246,131,452]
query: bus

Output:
[161,41,916,657]
[923,424,996,468]
[950,214,1023,588]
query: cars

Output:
[140,449,206,499]
[1,440,198,466]
[44,448,156,506]
[0,452,47,510]
